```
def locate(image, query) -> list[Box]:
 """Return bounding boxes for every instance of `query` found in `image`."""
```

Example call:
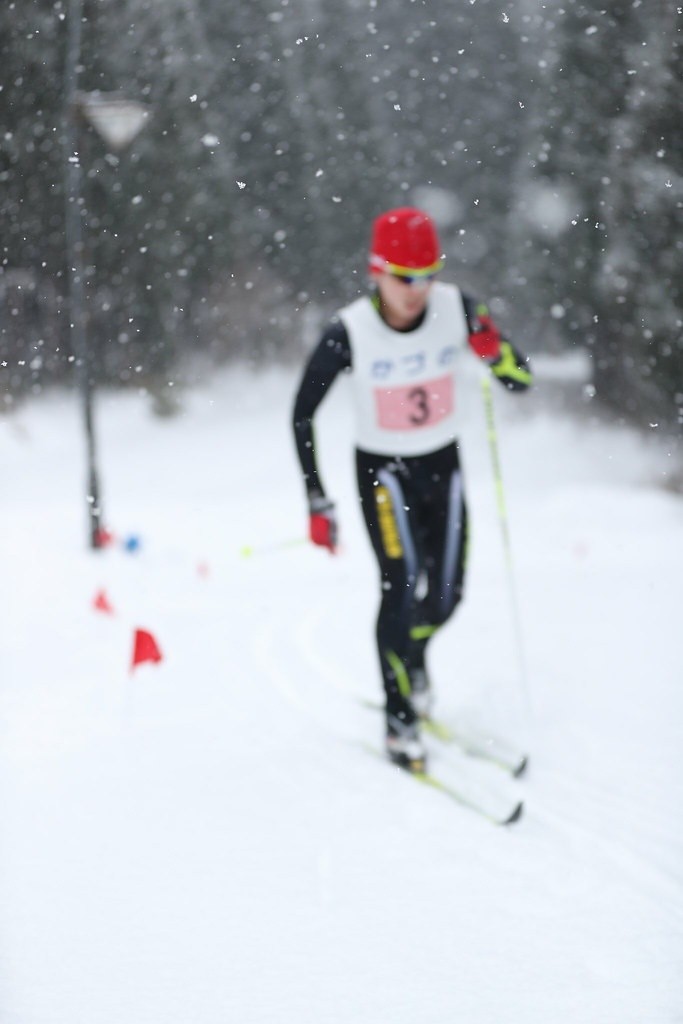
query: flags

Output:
[133,627,160,665]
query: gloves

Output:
[469,316,500,361]
[311,509,337,553]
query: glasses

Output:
[394,263,440,284]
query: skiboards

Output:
[352,693,532,827]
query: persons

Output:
[295,208,534,774]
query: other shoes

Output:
[408,668,431,714]
[387,705,424,767]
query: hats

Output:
[371,210,436,275]
